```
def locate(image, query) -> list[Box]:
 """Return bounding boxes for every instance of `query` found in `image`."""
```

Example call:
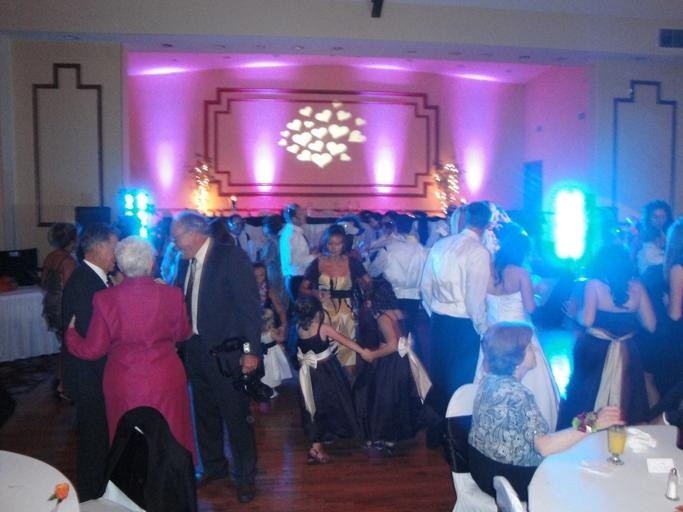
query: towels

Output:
[622,423,656,455]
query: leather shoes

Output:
[237,478,255,502]
[195,467,227,487]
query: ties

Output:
[186,258,196,321]
[236,237,240,246]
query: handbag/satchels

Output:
[214,336,245,375]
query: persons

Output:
[466,321,626,498]
[57,222,122,489]
[41,200,683,466]
[168,213,263,504]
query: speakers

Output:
[74,206,110,229]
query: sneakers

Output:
[232,373,273,400]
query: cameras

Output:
[233,368,274,403]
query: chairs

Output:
[442,382,518,512]
[77,426,177,512]
[493,475,530,512]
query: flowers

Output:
[45,481,70,504]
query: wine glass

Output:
[607,423,627,463]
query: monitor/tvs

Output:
[1,249,39,280]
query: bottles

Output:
[665,468,680,501]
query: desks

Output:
[0,283,66,363]
